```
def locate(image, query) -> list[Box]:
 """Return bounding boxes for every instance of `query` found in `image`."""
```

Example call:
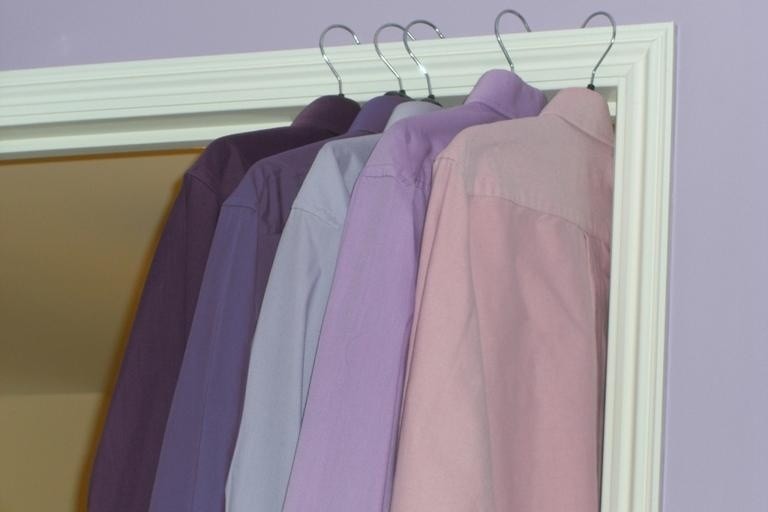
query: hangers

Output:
[319,7,617,106]
